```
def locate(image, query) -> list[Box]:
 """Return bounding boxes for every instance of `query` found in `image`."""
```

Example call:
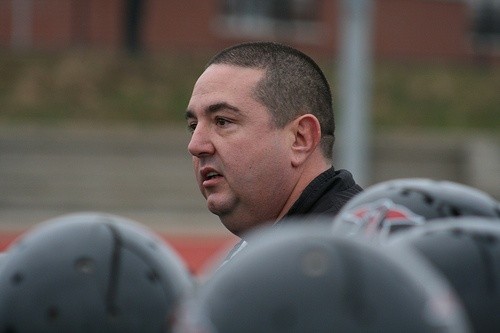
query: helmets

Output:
[2,212,189,333]
[181,218,469,330]
[335,177,498,239]
[392,213,499,333]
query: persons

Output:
[184,41,364,240]
